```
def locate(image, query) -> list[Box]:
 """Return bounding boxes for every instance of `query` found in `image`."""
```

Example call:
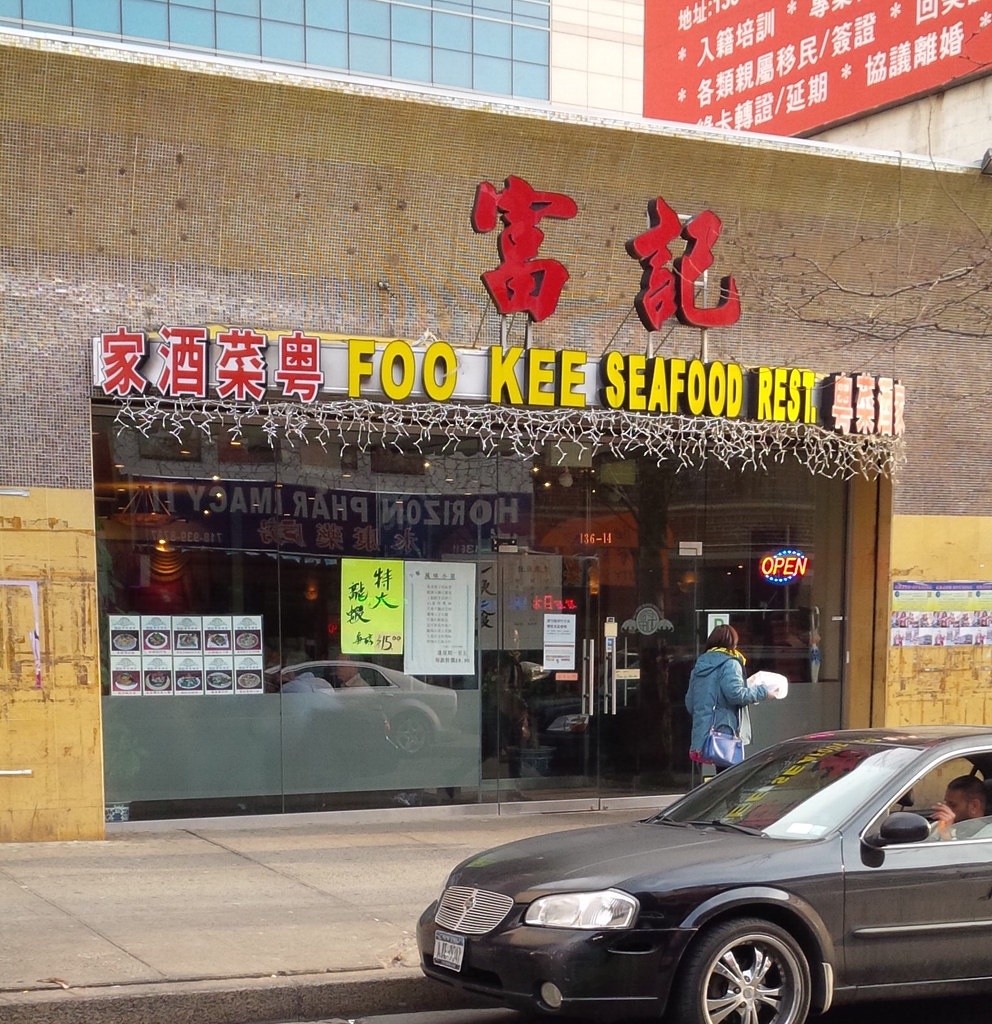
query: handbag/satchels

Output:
[702,723,745,768]
[495,685,531,747]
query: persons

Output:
[925,775,992,842]
[684,624,778,784]
[278,652,333,691]
[334,651,369,687]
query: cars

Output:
[415,723,992,1024]
[266,661,457,758]
